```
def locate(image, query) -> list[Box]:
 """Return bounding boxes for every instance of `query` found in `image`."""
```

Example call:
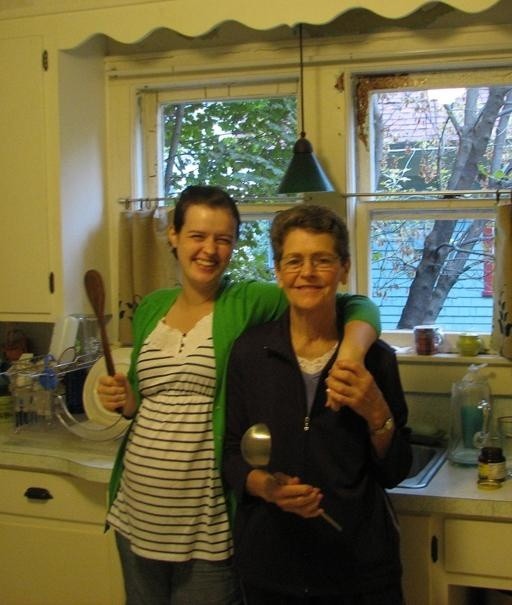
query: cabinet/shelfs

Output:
[0,460,126,604]
[431,514,512,605]
[0,14,114,329]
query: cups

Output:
[412,325,442,355]
[456,332,481,357]
[496,416,511,459]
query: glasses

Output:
[281,255,342,274]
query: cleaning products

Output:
[458,361,491,449]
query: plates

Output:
[82,346,138,429]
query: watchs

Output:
[369,415,394,436]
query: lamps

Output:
[272,19,338,198]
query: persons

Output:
[94,184,381,605]
[221,204,414,605]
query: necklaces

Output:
[181,284,219,304]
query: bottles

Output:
[11,373,52,427]
[445,363,500,467]
[475,445,507,485]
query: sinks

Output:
[395,439,448,490]
